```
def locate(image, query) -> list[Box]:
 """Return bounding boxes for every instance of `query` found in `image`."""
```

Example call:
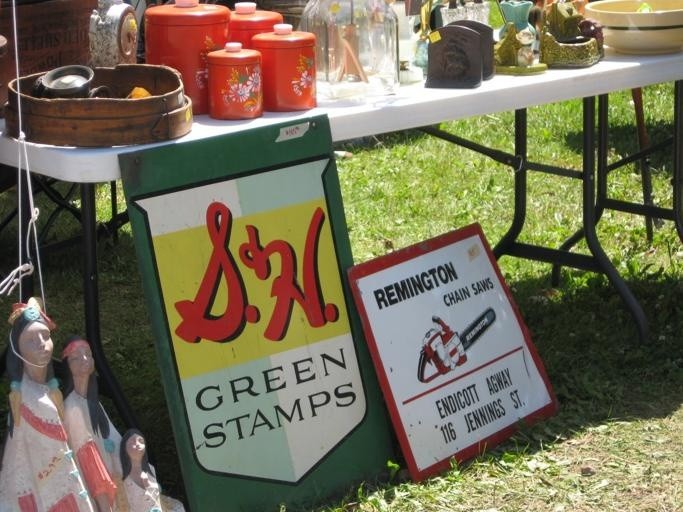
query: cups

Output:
[144,0,316,119]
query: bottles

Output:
[300,0,400,105]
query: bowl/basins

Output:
[585,0,683,54]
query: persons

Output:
[1,298,97,512]
[119,430,184,511]
[57,340,125,511]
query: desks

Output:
[0,50,683,485]
